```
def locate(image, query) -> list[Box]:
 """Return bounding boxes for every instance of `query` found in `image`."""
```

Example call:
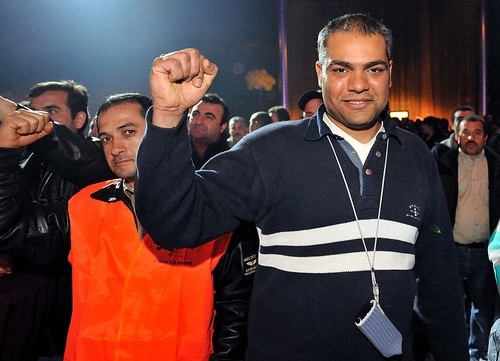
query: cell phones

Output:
[355,301,402,357]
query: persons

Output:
[134,13,470,361]
[0,79,500,361]
